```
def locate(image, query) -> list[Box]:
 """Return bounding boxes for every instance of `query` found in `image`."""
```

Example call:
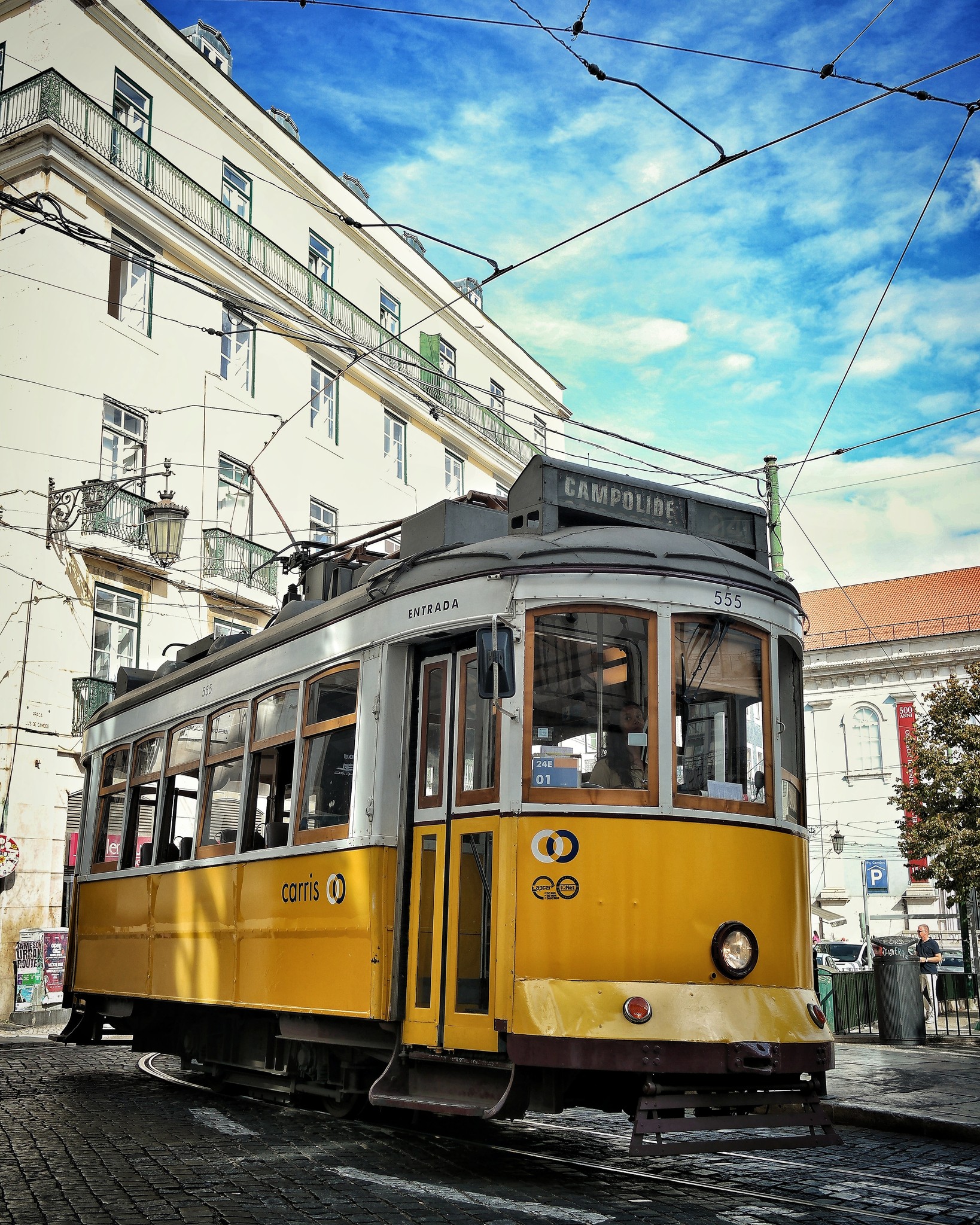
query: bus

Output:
[49,454,836,1118]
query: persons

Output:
[812,930,820,942]
[589,702,648,789]
[915,924,941,1024]
[812,940,819,951]
[817,948,821,953]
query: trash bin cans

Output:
[817,964,839,1033]
[870,936,926,1046]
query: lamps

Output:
[807,819,844,855]
[46,458,188,567]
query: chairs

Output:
[140,816,349,866]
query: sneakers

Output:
[925,1016,936,1024]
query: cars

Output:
[813,940,882,972]
[938,949,965,973]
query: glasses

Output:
[917,930,925,932]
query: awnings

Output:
[811,904,847,927]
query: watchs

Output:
[925,957,928,962]
